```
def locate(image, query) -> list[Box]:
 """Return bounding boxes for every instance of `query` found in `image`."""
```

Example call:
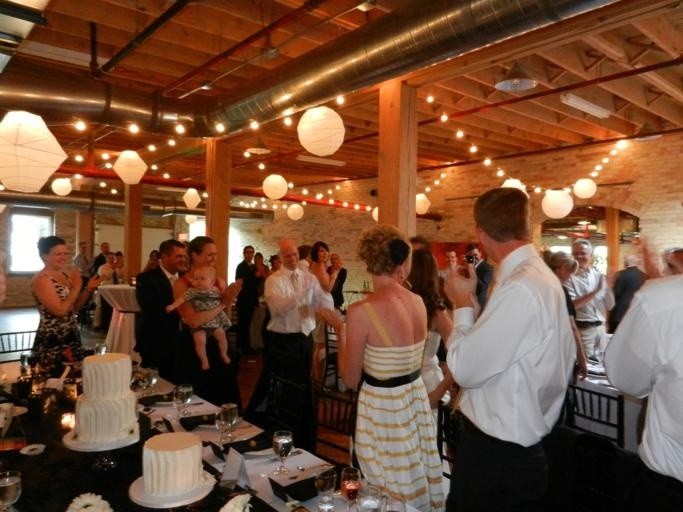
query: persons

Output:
[31,236,104,365]
[408,234,681,363]
[163,266,232,372]
[310,224,452,511]
[172,236,244,430]
[68,239,349,316]
[250,240,326,452]
[127,239,186,384]
[597,268,680,510]
[436,188,581,512]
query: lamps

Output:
[181,186,201,209]
[245,137,272,157]
[261,173,304,221]
[493,60,537,93]
[0,111,149,198]
[501,176,598,220]
[297,106,345,160]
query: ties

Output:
[290,274,313,337]
[112,272,118,284]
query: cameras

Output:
[465,255,475,265]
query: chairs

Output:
[320,321,353,400]
[562,383,623,449]
[434,398,453,479]
[308,384,359,486]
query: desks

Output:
[97,285,140,358]
[573,364,649,450]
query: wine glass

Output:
[593,335,608,369]
[176,385,193,416]
[20,352,32,368]
[95,342,108,355]
[221,404,239,439]
[145,369,159,394]
[273,431,293,475]
[340,467,361,512]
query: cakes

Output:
[140,432,203,496]
[75,352,138,440]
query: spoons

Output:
[297,463,333,471]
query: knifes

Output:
[256,450,302,466]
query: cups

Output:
[455,255,476,279]
[316,470,337,511]
[383,496,406,512]
[358,486,386,511]
[0,471,23,505]
